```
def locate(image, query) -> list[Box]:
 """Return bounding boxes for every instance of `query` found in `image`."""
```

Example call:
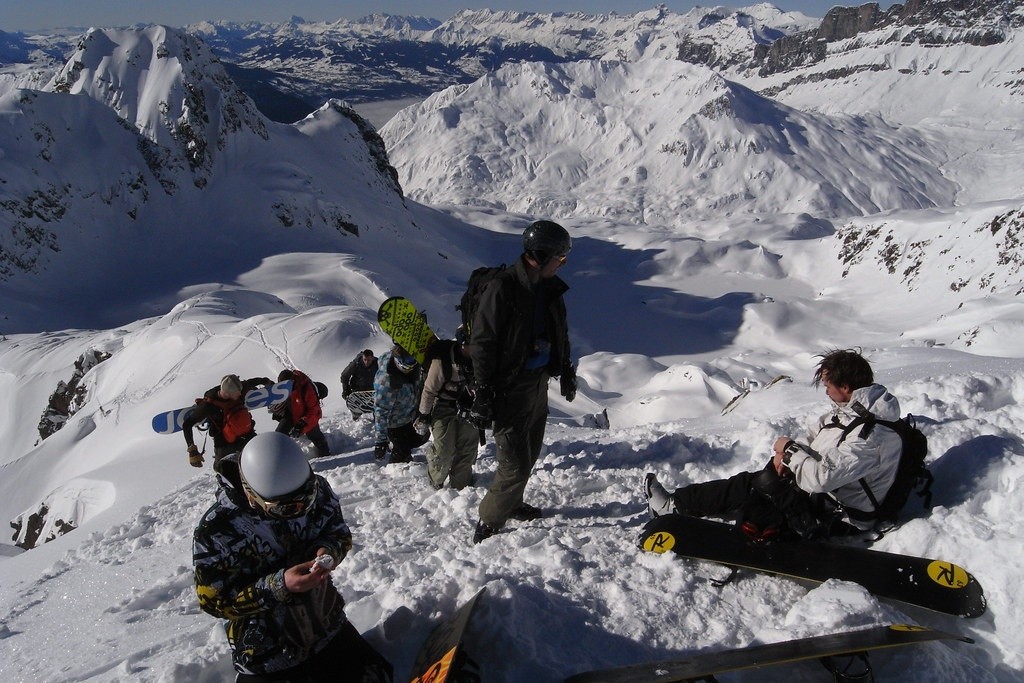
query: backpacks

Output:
[312,382,328,407]
[422,339,454,382]
[817,401,934,542]
[454,262,536,354]
[203,397,252,444]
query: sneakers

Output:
[509,502,542,522]
[473,518,499,545]
[643,473,680,521]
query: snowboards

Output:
[375,293,440,372]
[633,512,989,620]
[566,623,977,683]
[151,379,294,435]
[346,390,375,414]
[408,584,490,683]
[314,381,328,398]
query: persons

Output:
[274,370,330,458]
[341,349,380,423]
[182,375,276,468]
[372,322,480,491]
[192,431,396,683]
[466,219,579,542]
[643,349,903,536]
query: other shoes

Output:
[428,470,443,491]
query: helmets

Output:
[392,345,417,374]
[237,432,318,521]
[522,220,572,269]
[737,495,783,545]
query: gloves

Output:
[342,385,352,400]
[560,372,578,402]
[413,413,432,436]
[374,434,391,461]
[465,399,492,430]
[288,422,304,438]
[186,445,205,468]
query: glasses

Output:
[551,257,567,265]
[238,451,317,520]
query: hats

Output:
[278,369,293,383]
[220,374,243,393]
